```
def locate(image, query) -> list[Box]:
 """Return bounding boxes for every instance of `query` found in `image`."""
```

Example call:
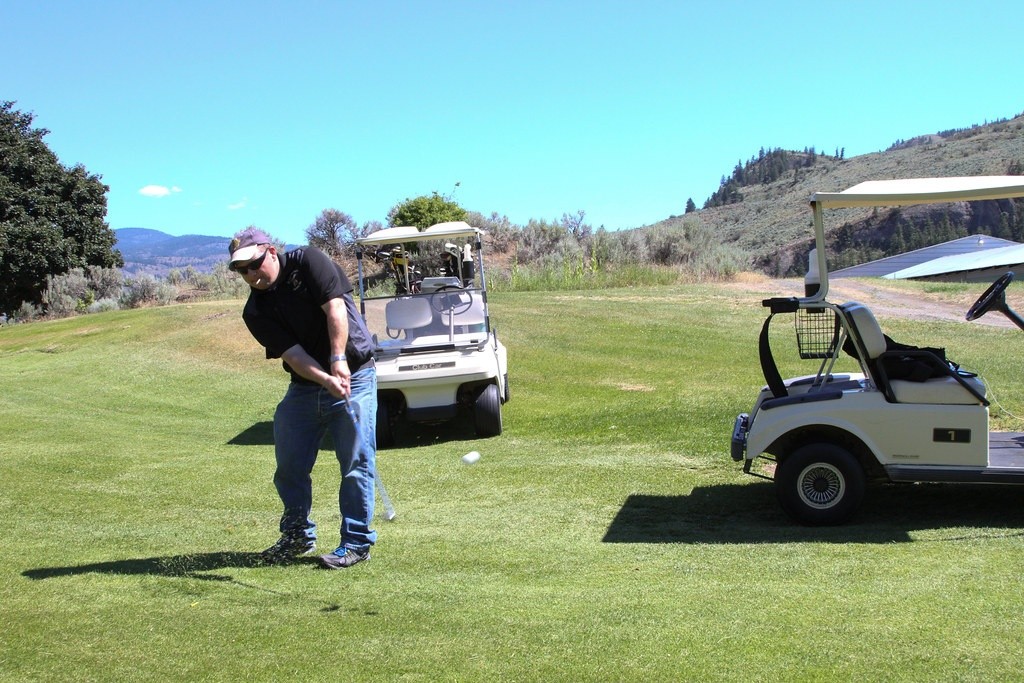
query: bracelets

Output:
[330,355,347,362]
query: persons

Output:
[228,225,377,570]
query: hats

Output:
[228,226,272,271]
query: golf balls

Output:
[461,450,479,463]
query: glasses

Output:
[233,248,269,274]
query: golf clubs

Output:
[343,391,396,524]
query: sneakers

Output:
[260,536,316,562]
[319,546,371,570]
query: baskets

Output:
[795,308,840,359]
